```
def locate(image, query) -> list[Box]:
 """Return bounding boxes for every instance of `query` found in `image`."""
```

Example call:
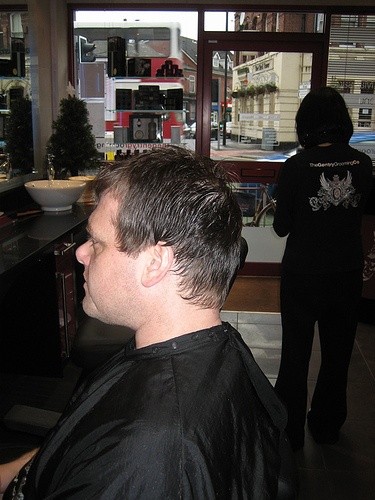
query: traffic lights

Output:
[78,35,96,64]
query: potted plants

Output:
[230,81,278,98]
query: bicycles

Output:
[229,182,277,228]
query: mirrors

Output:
[0,0,38,193]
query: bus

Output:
[73,20,187,154]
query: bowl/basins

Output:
[24,179,86,212]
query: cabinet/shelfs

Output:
[104,73,187,158]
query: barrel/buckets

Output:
[68,175,96,203]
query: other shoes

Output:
[307,408,337,444]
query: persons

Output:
[0,145,280,500]
[274,87,375,437]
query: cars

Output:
[189,120,218,140]
